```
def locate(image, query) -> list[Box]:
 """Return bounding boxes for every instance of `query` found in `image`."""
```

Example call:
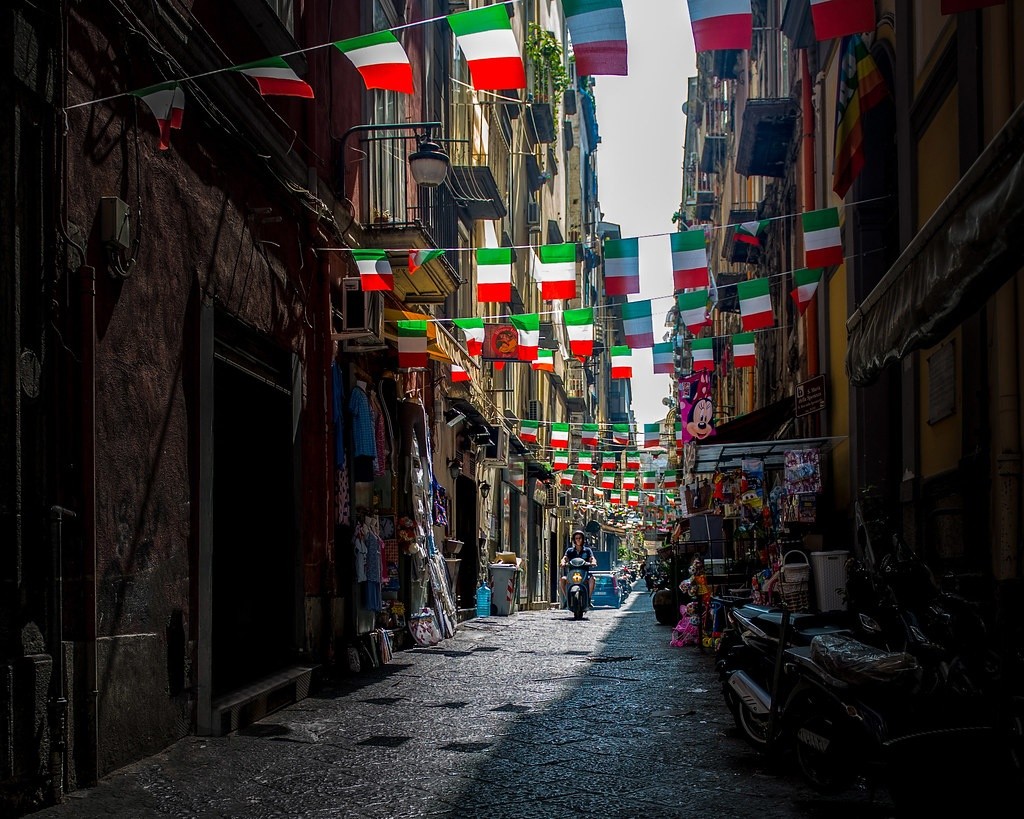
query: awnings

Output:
[691,436,846,473]
[677,393,797,479]
[843,103,1024,389]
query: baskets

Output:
[783,550,810,582]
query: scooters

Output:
[558,558,597,620]
[709,538,1024,798]
[616,559,667,602]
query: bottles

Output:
[476,582,492,618]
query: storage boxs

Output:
[783,550,810,582]
[777,582,809,611]
[811,549,849,612]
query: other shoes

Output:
[563,601,567,609]
[588,597,593,609]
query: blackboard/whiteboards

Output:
[794,374,826,418]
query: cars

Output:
[590,573,623,609]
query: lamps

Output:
[446,457,463,484]
[478,480,491,500]
[341,121,450,187]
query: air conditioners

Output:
[527,202,541,234]
[544,485,558,509]
[332,275,388,351]
[477,423,509,468]
[558,491,571,509]
[562,503,574,521]
[528,399,544,427]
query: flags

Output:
[229,56,316,99]
[445,331,761,384]
[688,0,753,52]
[130,79,186,149]
[351,208,848,299]
[448,3,528,90]
[831,34,893,200]
[519,416,722,508]
[333,29,416,94]
[562,0,628,76]
[810,0,878,42]
[396,267,827,368]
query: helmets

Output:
[572,530,585,543]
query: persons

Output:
[640,562,646,579]
[349,371,432,610]
[624,566,630,574]
[560,530,597,608]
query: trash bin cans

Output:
[489,565,519,616]
